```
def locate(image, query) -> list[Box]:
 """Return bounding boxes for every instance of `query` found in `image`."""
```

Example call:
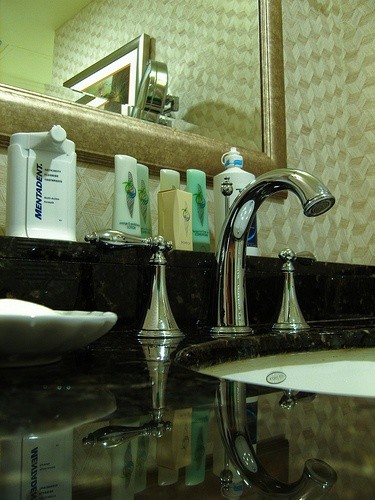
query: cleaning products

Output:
[212,147,259,256]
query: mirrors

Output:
[0,0,291,200]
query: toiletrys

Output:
[112,155,142,243]
[0,405,244,500]
[186,168,210,251]
[134,163,153,243]
[160,169,180,250]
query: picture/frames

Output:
[62,32,152,108]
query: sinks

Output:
[176,326,375,400]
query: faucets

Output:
[220,379,339,500]
[210,169,336,335]
